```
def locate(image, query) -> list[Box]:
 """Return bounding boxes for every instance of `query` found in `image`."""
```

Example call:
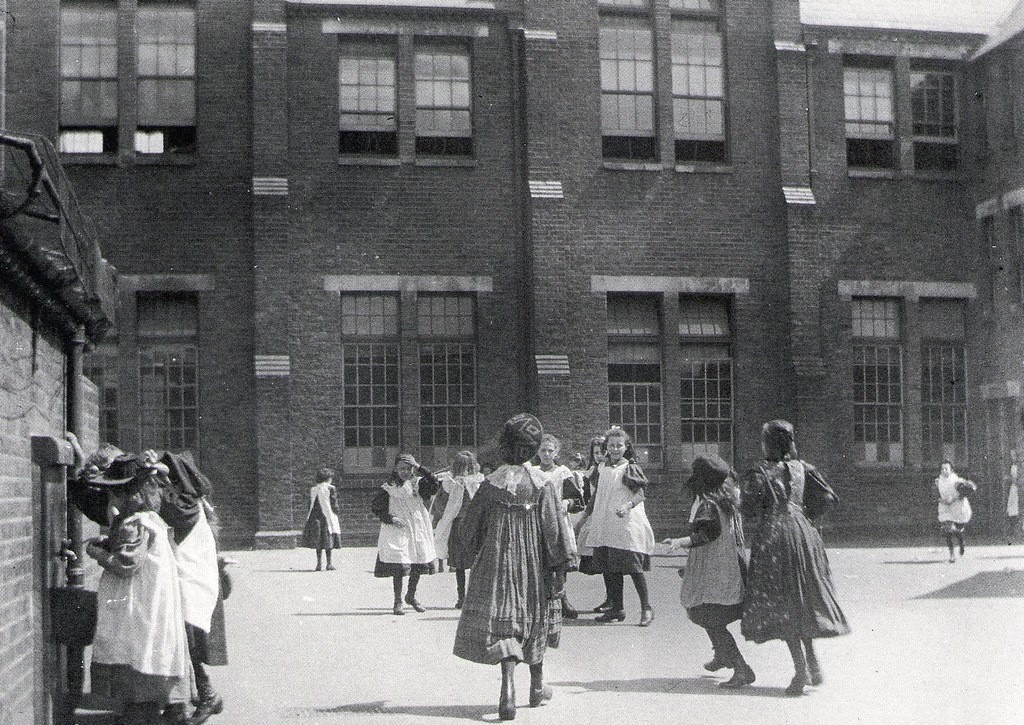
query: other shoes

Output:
[786,672,807,697]
[594,602,613,612]
[562,598,578,620]
[949,555,955,563]
[595,610,625,622]
[640,609,655,626]
[959,542,965,555]
[719,667,756,689]
[808,653,822,686]
[704,656,733,672]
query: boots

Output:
[498,657,516,720]
[529,663,552,707]
[405,574,425,612]
[326,548,335,571]
[181,673,224,724]
[454,568,466,609]
[316,551,322,570]
[392,581,404,615]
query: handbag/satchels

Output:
[801,464,841,522]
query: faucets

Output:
[58,537,78,562]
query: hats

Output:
[89,455,153,486]
[498,412,542,466]
[692,453,729,485]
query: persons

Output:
[1005,448,1019,534]
[733,419,851,696]
[371,451,440,615]
[302,468,342,571]
[454,412,582,721]
[663,453,756,689]
[74,443,238,725]
[574,425,655,627]
[534,434,613,618]
[934,460,978,563]
[429,451,485,609]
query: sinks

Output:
[49,585,100,647]
